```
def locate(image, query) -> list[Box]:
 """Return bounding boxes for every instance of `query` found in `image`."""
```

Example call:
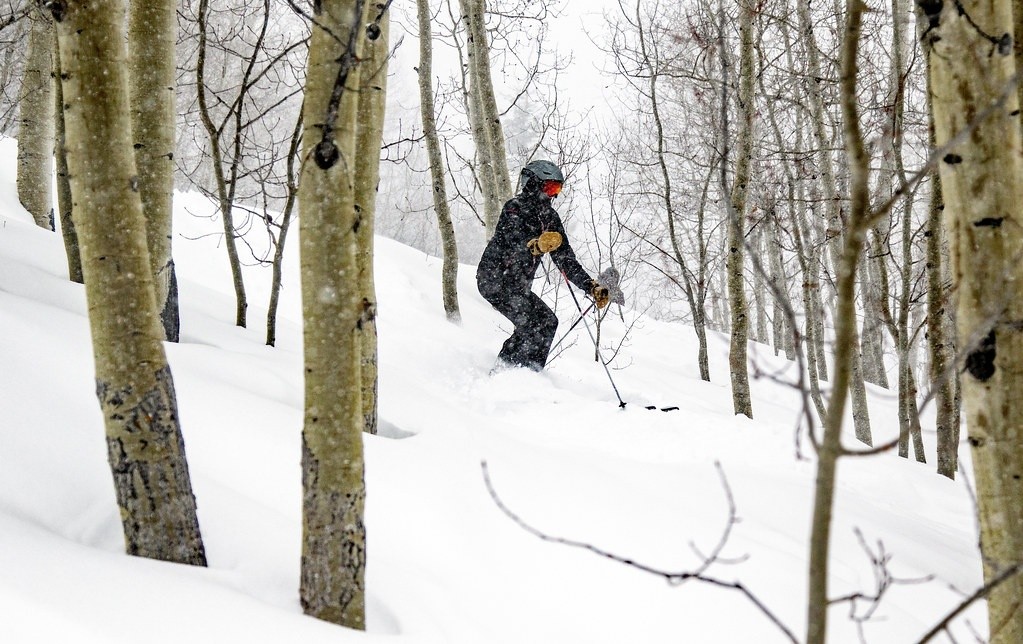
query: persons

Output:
[597,266,626,307]
[476,161,609,372]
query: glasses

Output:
[542,180,563,198]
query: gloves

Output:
[526,232,562,256]
[592,285,609,308]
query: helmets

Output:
[521,160,564,197]
